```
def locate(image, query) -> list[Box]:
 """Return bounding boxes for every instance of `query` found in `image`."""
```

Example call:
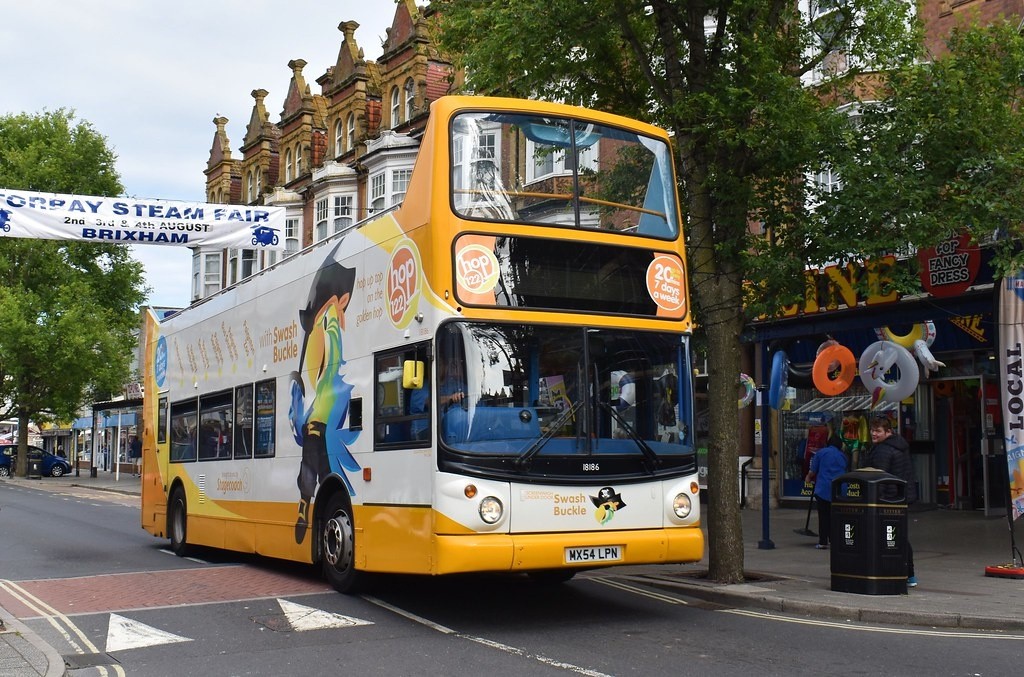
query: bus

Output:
[141,94,705,595]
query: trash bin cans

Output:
[830,467,910,596]
[28,453,42,480]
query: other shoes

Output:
[815,545,828,550]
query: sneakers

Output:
[906,576,917,586]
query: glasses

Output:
[876,414,891,428]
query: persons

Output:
[803,411,833,482]
[130,436,142,477]
[805,436,847,549]
[869,417,918,586]
[128,435,134,462]
[840,415,869,469]
[58,445,66,458]
[410,356,486,441]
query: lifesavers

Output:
[812,346,856,396]
[874,319,947,379]
[859,340,919,412]
[933,377,951,397]
[737,372,756,410]
[816,340,840,372]
[768,350,789,411]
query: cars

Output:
[0,444,73,477]
